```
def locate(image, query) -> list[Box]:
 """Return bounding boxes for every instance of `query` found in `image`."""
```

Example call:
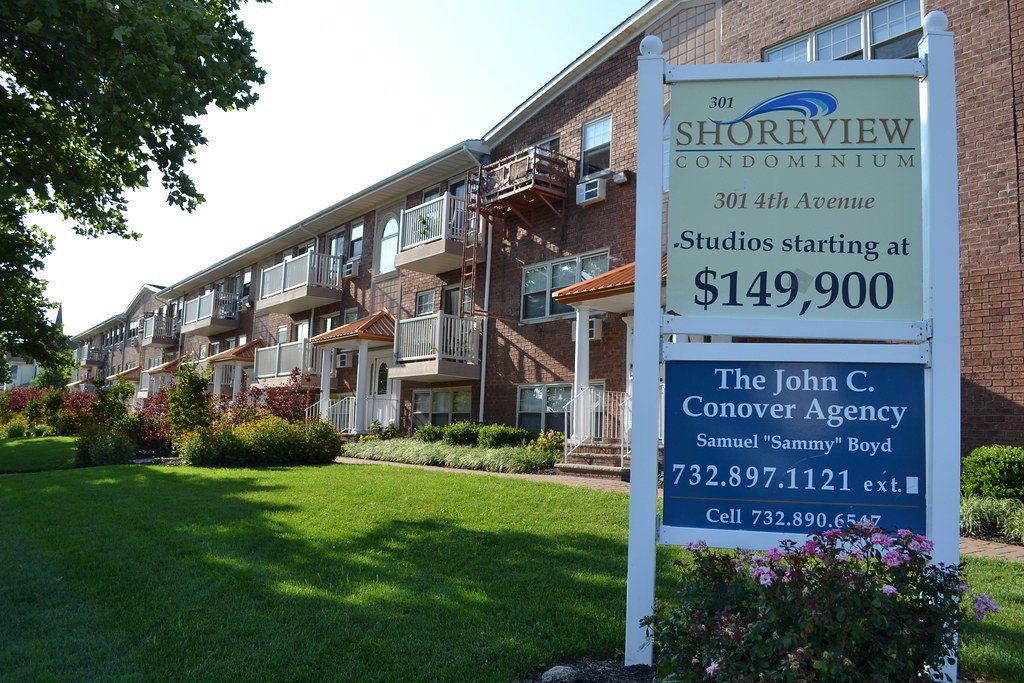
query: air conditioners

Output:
[336,353,353,369]
[575,178,607,208]
[173,324,180,334]
[342,261,359,279]
[572,319,603,342]
[236,301,247,314]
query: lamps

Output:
[612,170,632,186]
[130,339,138,347]
[245,301,254,308]
